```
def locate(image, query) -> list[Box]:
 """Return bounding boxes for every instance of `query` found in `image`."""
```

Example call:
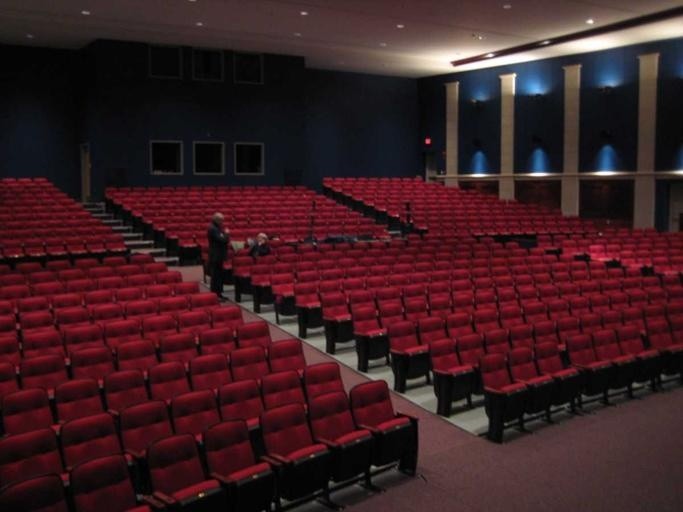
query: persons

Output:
[206,213,230,301]
[249,234,268,257]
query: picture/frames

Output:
[144,43,265,85]
[148,140,265,178]
